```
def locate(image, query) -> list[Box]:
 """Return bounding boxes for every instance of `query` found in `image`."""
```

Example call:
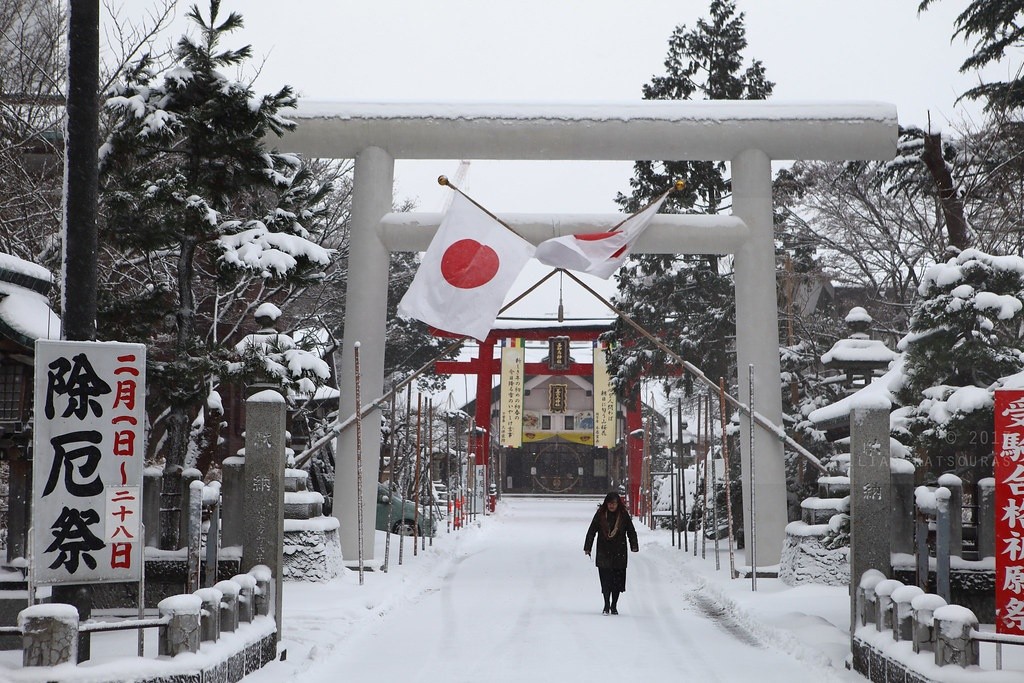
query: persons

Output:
[583,492,639,616]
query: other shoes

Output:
[603,605,610,616]
[611,604,618,615]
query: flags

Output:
[535,190,666,282]
[396,189,536,344]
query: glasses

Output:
[608,502,618,505]
